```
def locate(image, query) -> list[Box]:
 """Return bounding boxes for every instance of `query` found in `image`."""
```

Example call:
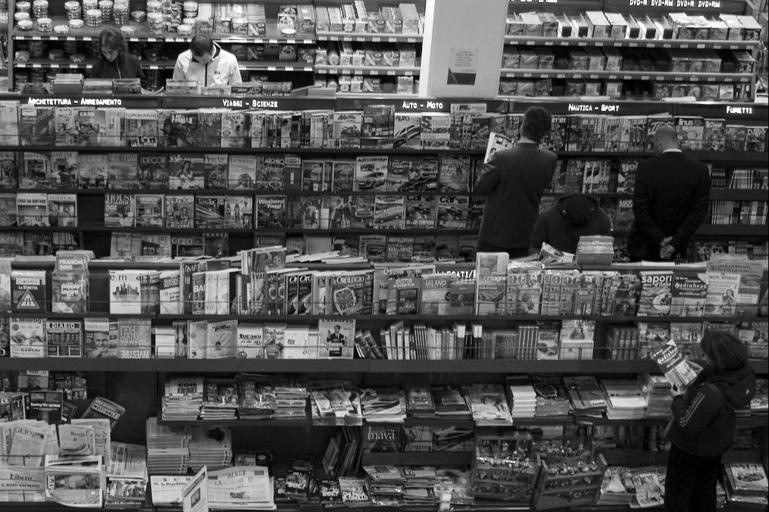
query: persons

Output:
[86,25,145,89]
[162,28,246,93]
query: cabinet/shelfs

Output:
[9,1,316,91]
[512,102,768,240]
[318,1,764,84]
[0,261,769,512]
[0,96,510,237]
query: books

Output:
[1,109,768,511]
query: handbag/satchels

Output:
[660,417,676,466]
